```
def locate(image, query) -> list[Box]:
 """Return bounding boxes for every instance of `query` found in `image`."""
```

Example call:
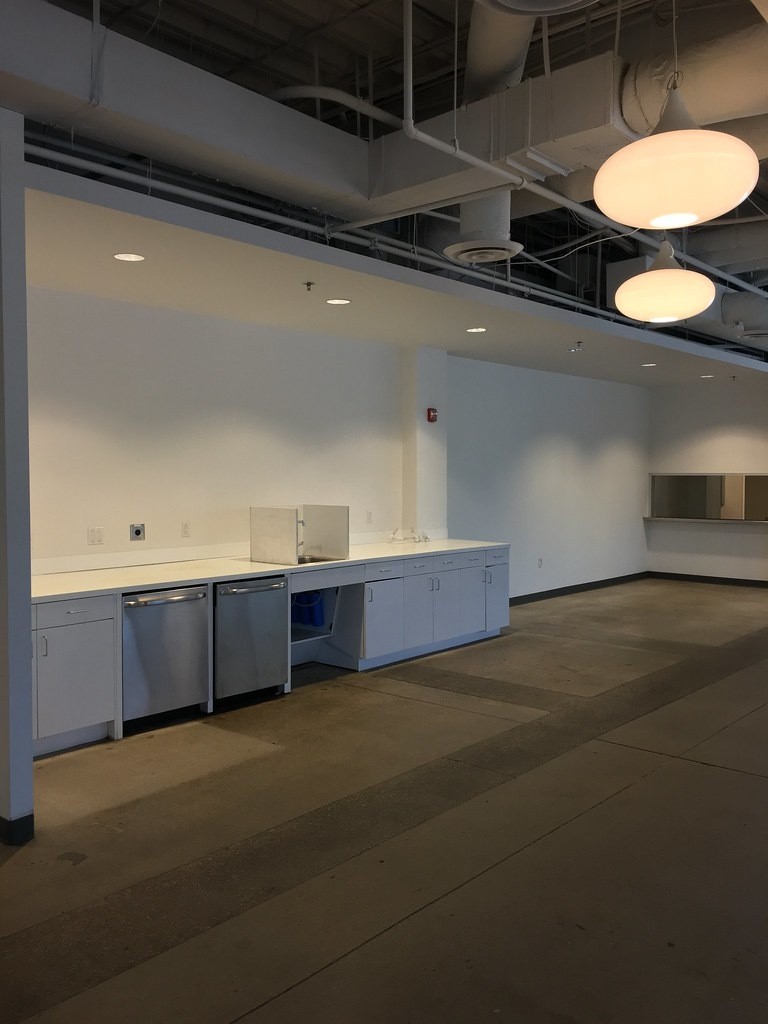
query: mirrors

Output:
[648,473,768,521]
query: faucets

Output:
[297,519,306,527]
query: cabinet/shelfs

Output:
[461,548,509,644]
[291,560,404,671]
[29,594,118,757]
[405,553,461,660]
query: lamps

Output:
[614,230,716,323]
[595,0,760,230]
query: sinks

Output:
[297,554,347,565]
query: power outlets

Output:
[87,526,104,544]
